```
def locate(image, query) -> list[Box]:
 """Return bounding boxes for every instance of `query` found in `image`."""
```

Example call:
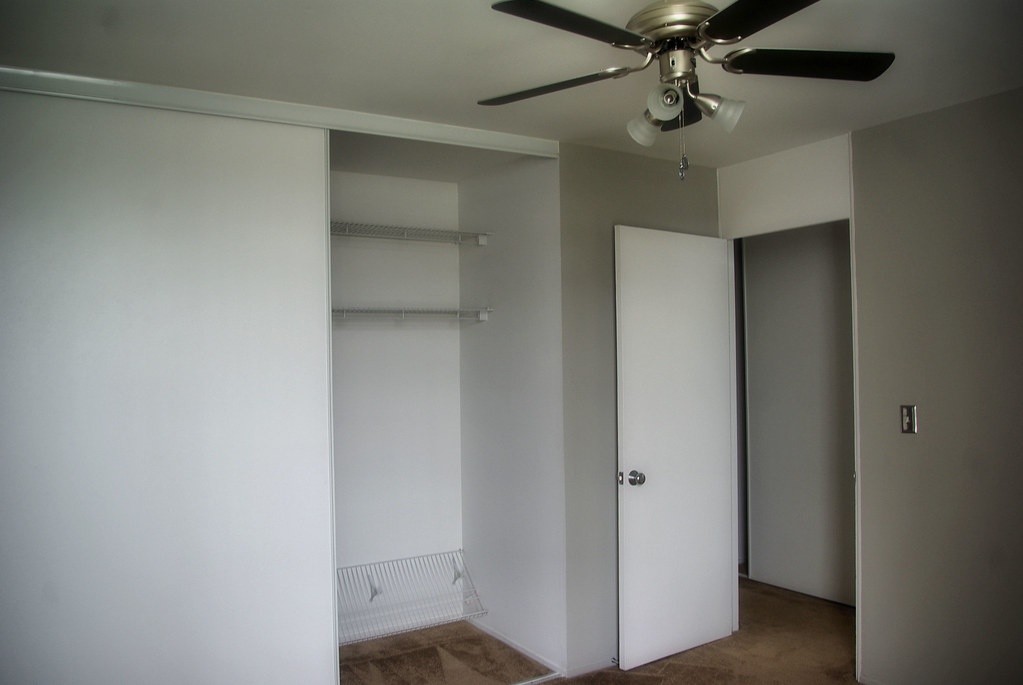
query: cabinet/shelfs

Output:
[330,221,495,329]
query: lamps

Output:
[629,49,746,148]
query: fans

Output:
[476,0,896,133]
